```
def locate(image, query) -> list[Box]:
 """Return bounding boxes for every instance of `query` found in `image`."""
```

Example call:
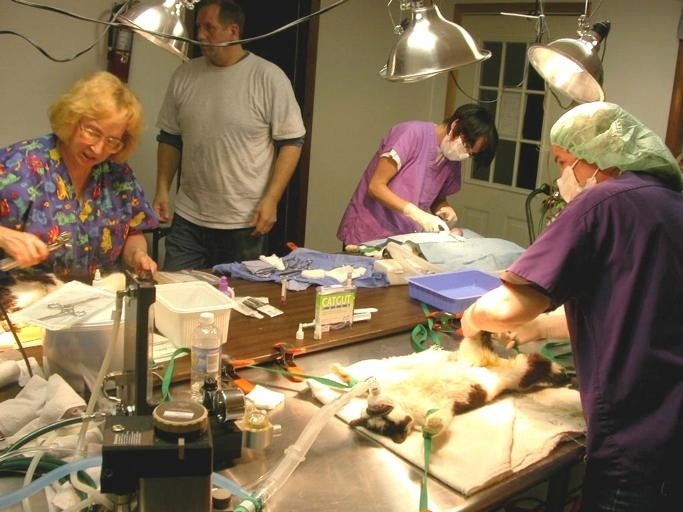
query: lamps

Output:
[527,19,611,103]
[378,0,492,85]
[113,0,198,65]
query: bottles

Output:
[188,311,222,404]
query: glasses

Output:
[77,118,124,155]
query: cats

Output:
[342,227,465,259]
[1,266,124,319]
[330,331,570,445]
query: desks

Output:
[0,228,588,511]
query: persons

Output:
[459,100,682,512]
[0,69,161,280]
[336,102,501,250]
[149,0,307,271]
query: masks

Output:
[555,164,598,203]
[440,133,470,162]
